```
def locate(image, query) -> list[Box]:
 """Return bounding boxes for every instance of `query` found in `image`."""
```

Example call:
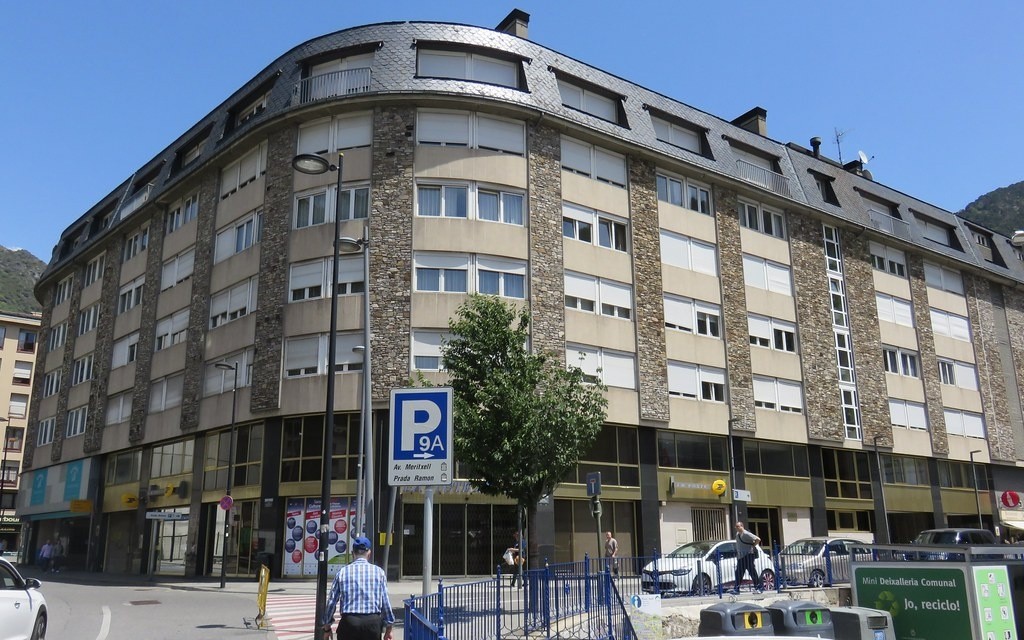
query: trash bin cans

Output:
[698,600,896,640]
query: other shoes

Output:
[753,590,763,594]
[729,589,740,595]
[511,584,515,587]
[522,584,525,586]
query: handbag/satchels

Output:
[515,556,525,565]
[503,550,514,565]
[752,547,759,559]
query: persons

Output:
[728,521,764,594]
[321,537,395,640]
[604,532,621,581]
[504,530,527,586]
[39,539,64,573]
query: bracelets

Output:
[322,626,331,633]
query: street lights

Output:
[287,150,346,640]
[969,450,985,530]
[0,416,11,510]
[350,345,371,540]
[872,434,890,545]
[726,416,744,538]
[214,357,241,589]
[330,218,376,565]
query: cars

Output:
[769,536,878,587]
[640,536,779,594]
[901,528,1006,561]
[0,556,48,640]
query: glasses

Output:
[736,525,741,528]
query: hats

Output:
[353,537,371,551]
